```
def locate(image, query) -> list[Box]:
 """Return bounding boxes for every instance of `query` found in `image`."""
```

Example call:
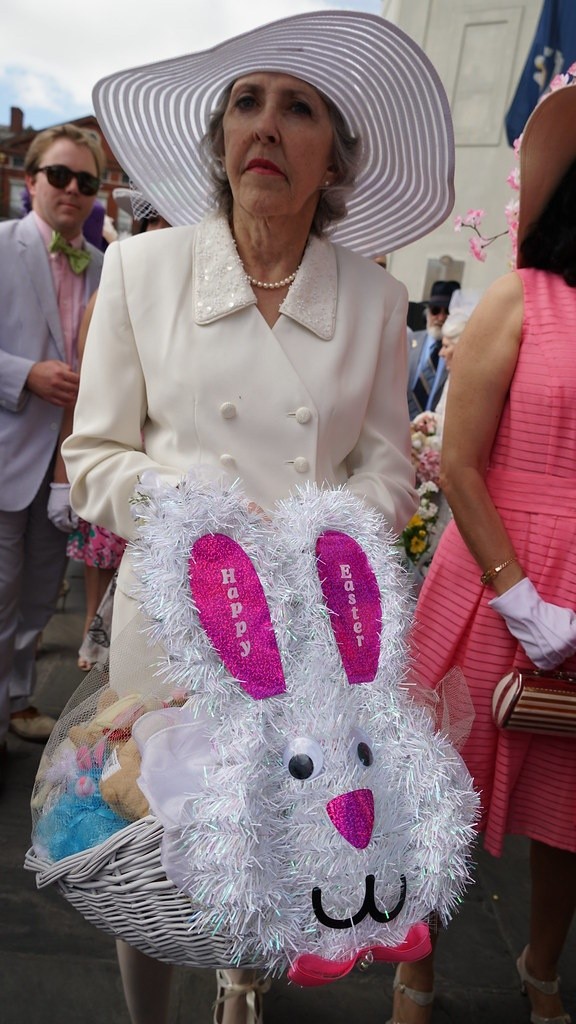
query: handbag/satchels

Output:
[491,659,576,740]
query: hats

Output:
[90,10,455,259]
[415,280,460,305]
[112,188,145,219]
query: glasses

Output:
[34,165,99,195]
[429,305,449,314]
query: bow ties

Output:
[47,232,91,273]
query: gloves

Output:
[489,576,576,670]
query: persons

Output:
[0,124,172,768]
[405,281,473,593]
[63,9,457,1024]
[390,86,575,1024]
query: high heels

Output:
[58,580,70,611]
[517,943,571,1023]
[391,960,436,1006]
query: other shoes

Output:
[212,969,269,1024]
[9,706,59,739]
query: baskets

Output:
[22,814,279,968]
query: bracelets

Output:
[481,557,518,584]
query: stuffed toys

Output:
[32,478,479,987]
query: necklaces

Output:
[246,265,301,289]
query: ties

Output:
[407,341,443,421]
